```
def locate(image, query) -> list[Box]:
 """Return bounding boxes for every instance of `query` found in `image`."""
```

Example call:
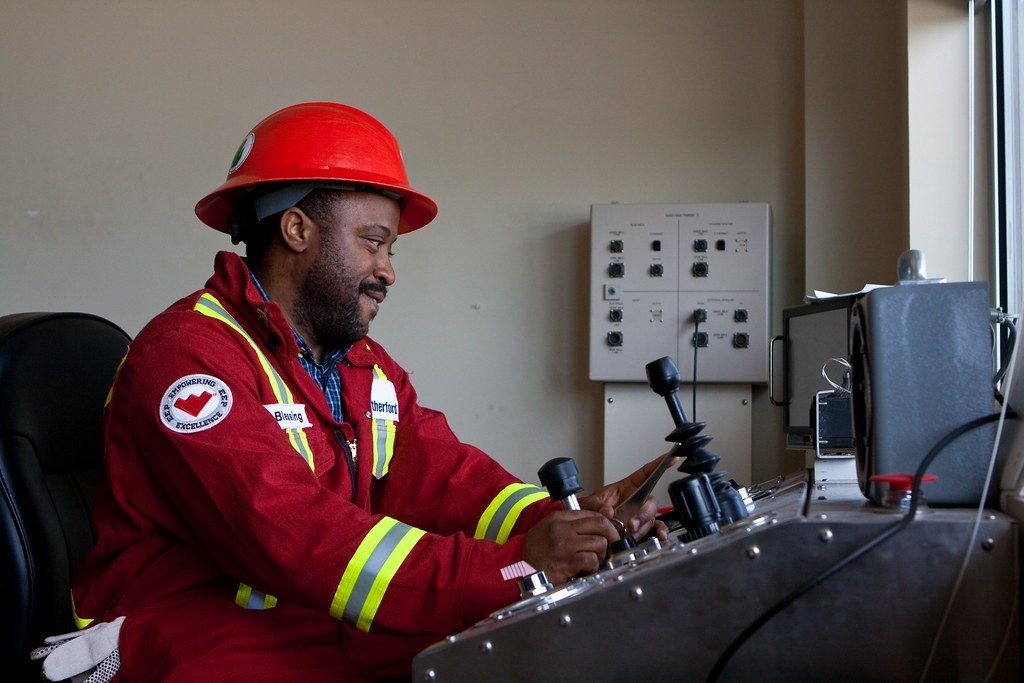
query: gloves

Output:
[30,613,127,683]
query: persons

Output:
[70,103,679,683]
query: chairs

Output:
[1,313,137,683]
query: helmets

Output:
[194,102,438,235]
